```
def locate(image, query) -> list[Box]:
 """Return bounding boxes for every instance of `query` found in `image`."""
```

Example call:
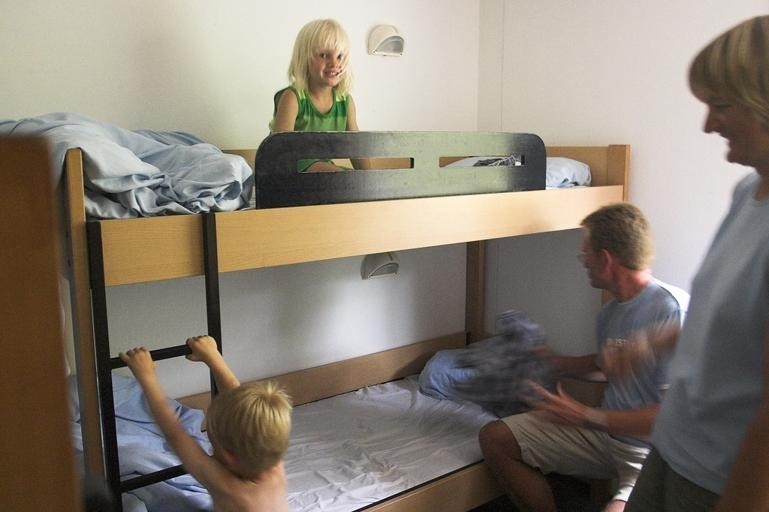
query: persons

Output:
[478,201,691,511]
[269,20,372,173]
[601,13,769,511]
[120,337,293,512]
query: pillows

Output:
[442,147,593,188]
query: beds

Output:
[62,130,630,511]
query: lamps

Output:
[366,23,406,55]
[361,251,401,279]
